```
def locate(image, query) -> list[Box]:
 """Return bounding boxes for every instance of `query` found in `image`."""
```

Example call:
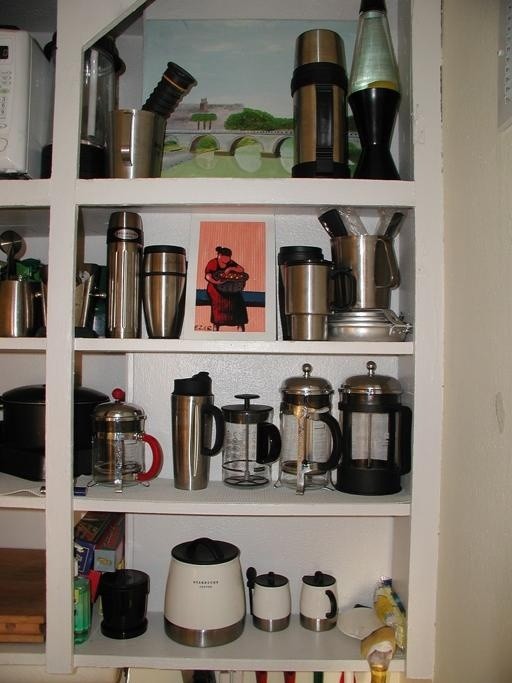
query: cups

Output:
[277,363,343,496]
[337,362,414,497]
[220,392,282,490]
[90,388,163,494]
[280,256,357,342]
[170,363,225,497]
[141,244,187,338]
[112,106,171,177]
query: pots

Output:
[0,376,111,429]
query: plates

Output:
[335,607,389,640]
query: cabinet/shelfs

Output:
[0,0,442,683]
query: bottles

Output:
[104,210,145,340]
[163,537,247,649]
[347,0,403,181]
[289,28,352,177]
[297,570,339,638]
[40,29,126,176]
[246,567,294,631]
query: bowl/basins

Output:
[329,310,411,342]
